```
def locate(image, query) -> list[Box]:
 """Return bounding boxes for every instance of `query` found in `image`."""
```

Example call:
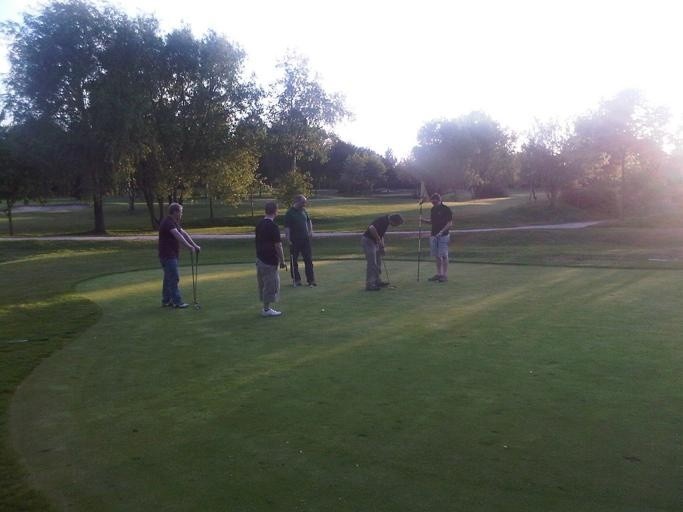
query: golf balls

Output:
[321,309,325,312]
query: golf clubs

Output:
[191,251,200,311]
[290,245,296,287]
[382,255,396,288]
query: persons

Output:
[421,193,452,281]
[158,203,201,308]
[361,215,403,291]
[256,201,286,316]
[284,195,318,286]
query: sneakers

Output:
[261,307,281,316]
[365,281,389,291]
[292,279,317,287]
[163,300,190,308]
[427,275,448,282]
